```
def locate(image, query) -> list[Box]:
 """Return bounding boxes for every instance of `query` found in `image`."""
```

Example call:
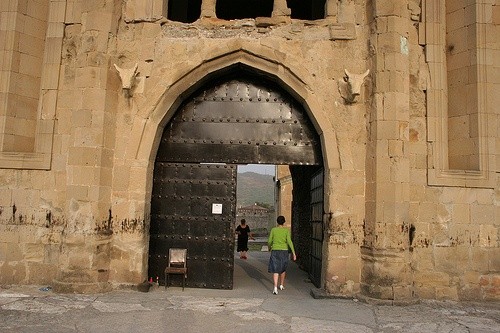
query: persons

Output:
[234,218,255,260]
[267,214,296,295]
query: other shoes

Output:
[243,256,247,259]
[240,256,243,259]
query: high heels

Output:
[272,287,280,296]
[279,285,285,291]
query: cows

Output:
[113,62,140,96]
[338,68,370,103]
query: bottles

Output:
[156,277,159,287]
[149,276,153,282]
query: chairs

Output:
[164,248,188,291]
